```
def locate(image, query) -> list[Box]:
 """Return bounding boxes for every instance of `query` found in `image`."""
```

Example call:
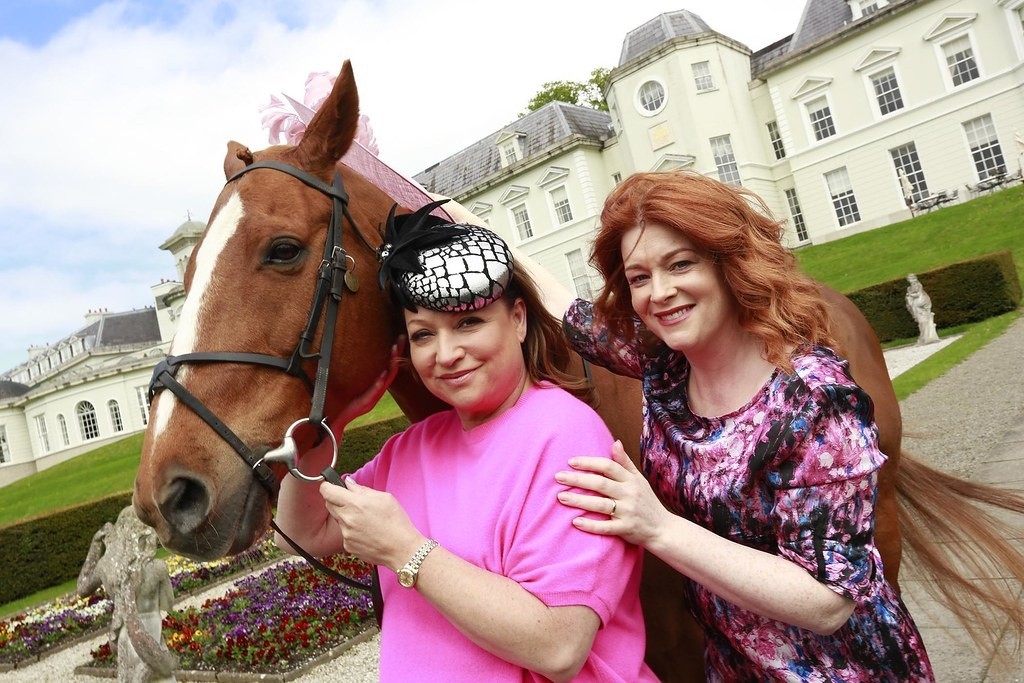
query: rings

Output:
[608,498,617,516]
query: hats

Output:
[372,198,516,315]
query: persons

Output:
[273,198,661,683]
[905,273,932,318]
[76,523,177,683]
[429,172,937,683]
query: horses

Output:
[134,60,1023,683]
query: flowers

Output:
[261,67,378,168]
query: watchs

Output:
[396,539,441,588]
